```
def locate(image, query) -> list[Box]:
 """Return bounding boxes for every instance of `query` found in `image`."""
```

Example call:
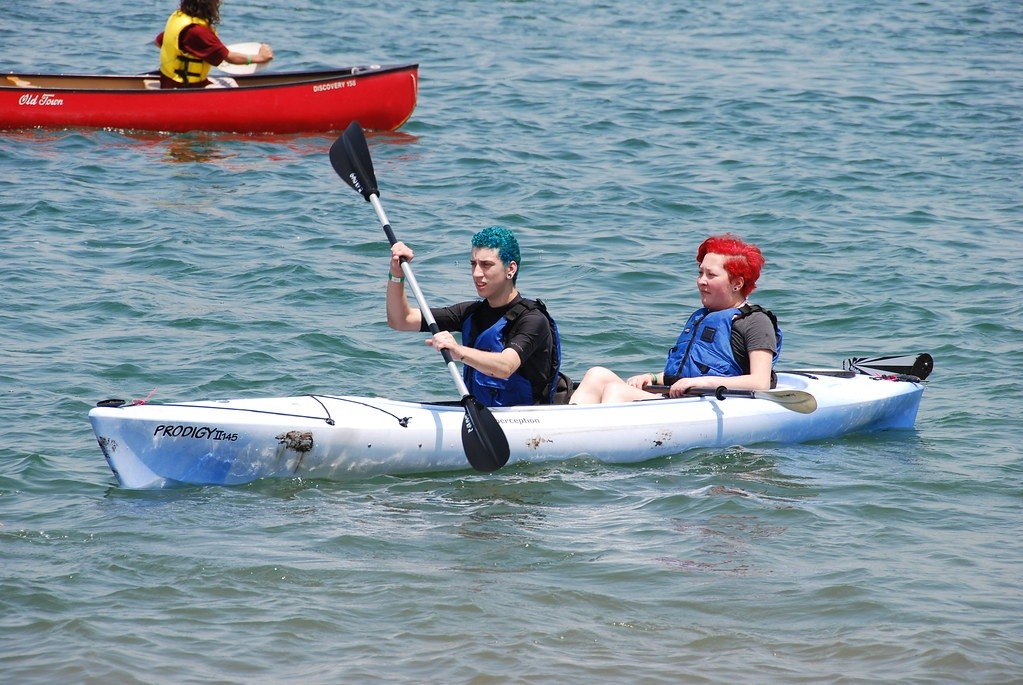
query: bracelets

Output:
[388,272,404,283]
[248,55,251,64]
[652,373,657,384]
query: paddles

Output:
[328,117,511,474]
[643,384,817,414]
[215,41,272,76]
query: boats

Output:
[0,61,419,134]
[88,354,936,492]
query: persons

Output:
[567,233,782,405]
[153,0,273,90]
[386,225,562,407]
